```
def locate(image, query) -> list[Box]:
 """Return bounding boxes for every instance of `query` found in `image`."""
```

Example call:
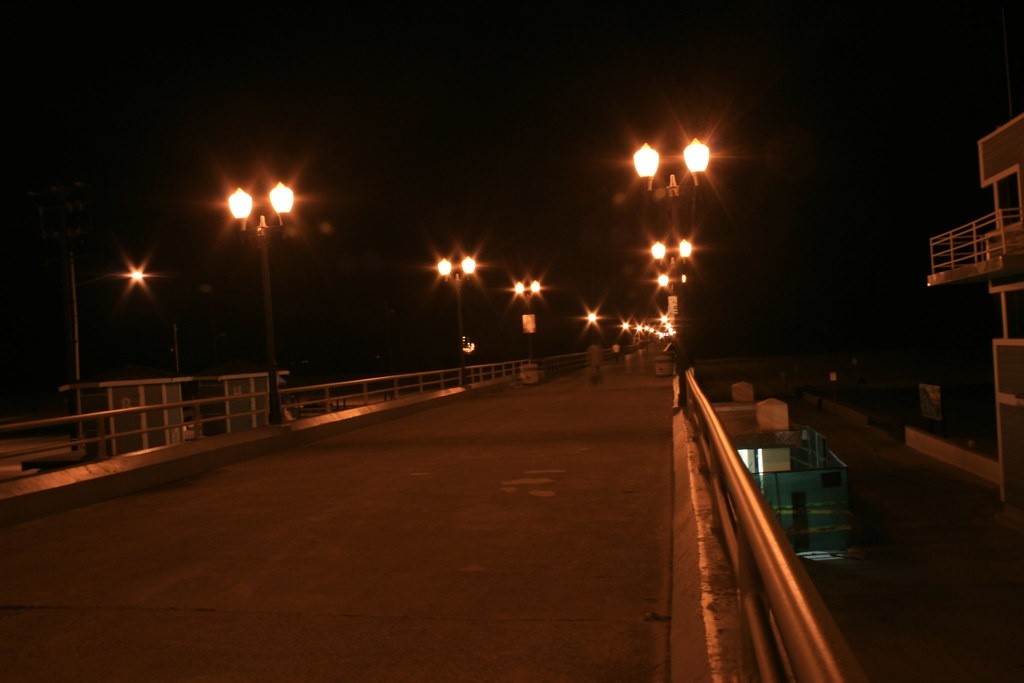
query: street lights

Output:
[227,180,297,424]
[650,239,694,412]
[437,254,475,387]
[514,280,541,363]
[633,137,711,418]
